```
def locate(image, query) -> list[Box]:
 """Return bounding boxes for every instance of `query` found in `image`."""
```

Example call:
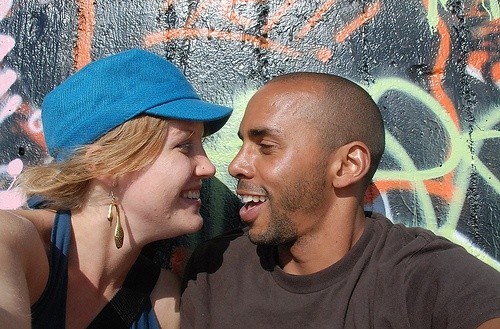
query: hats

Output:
[41,47,233,162]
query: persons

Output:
[0,49,216,329]
[178,71,500,329]
